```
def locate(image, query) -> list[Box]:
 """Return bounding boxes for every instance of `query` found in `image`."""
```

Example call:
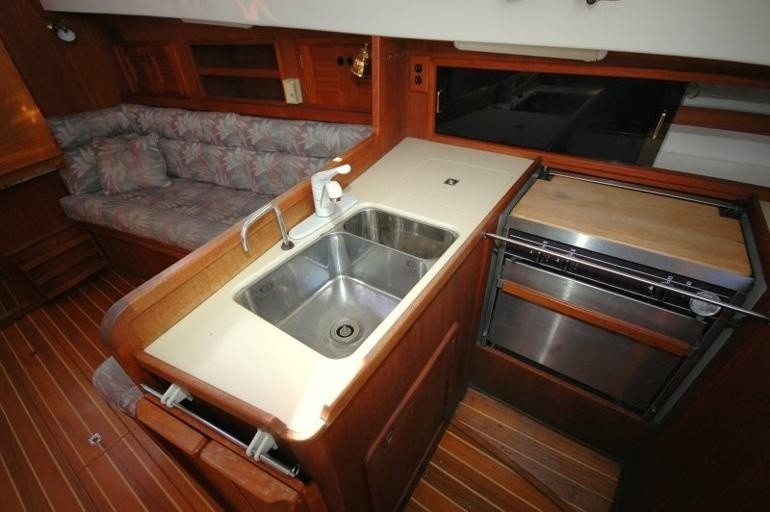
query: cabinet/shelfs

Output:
[310,235,492,512]
[405,47,766,200]
[116,40,371,112]
[0,172,107,334]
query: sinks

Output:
[334,203,458,264]
[231,231,429,362]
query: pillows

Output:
[92,132,172,196]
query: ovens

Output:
[476,165,766,424]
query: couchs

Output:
[47,104,374,277]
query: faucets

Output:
[239,200,297,254]
[288,162,362,239]
